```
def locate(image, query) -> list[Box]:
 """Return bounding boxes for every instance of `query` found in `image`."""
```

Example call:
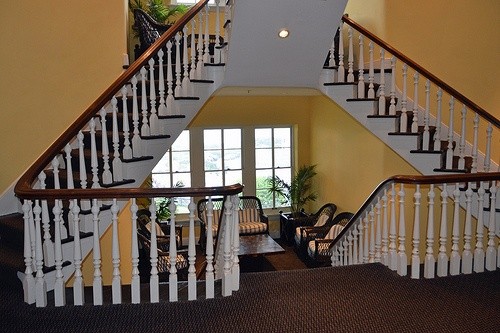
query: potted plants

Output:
[267,164,319,247]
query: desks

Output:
[187,33,224,63]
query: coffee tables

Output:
[213,233,285,272]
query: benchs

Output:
[197,196,269,253]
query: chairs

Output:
[138,214,183,250]
[304,212,354,268]
[134,9,184,64]
[292,203,337,259]
[137,228,188,281]
